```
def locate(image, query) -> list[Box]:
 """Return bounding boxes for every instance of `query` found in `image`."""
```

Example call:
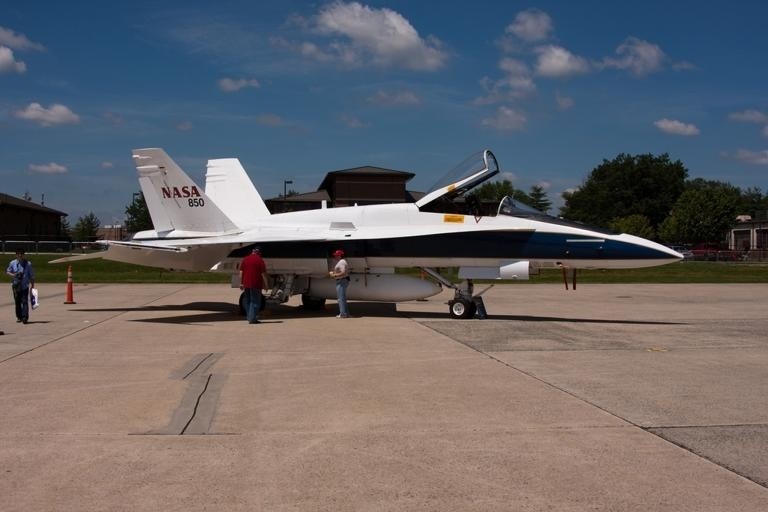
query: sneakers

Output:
[336,315,347,318]
[249,319,260,324]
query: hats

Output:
[333,250,344,256]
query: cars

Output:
[670,246,715,261]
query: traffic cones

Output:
[64,264,77,304]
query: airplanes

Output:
[47,147,685,318]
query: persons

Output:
[5,247,34,325]
[332,249,351,318]
[239,245,270,324]
